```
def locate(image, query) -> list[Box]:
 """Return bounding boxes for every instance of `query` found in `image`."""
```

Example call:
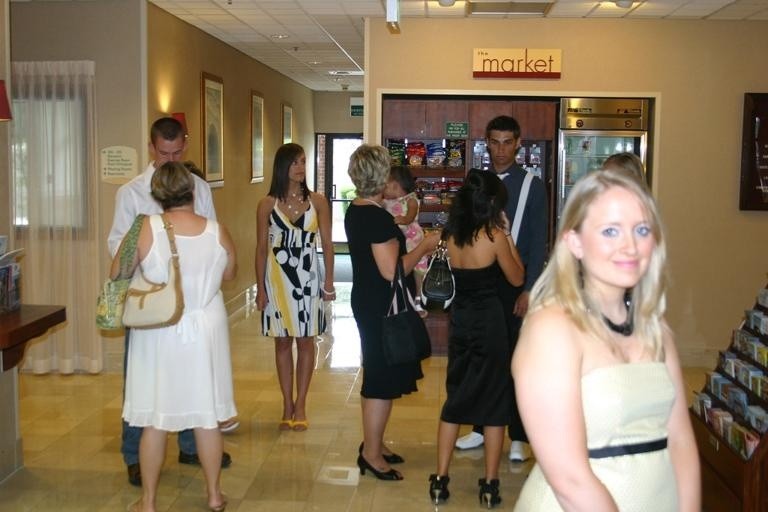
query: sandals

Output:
[412,294,430,320]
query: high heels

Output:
[292,407,309,433]
[357,452,404,483]
[359,440,408,464]
[478,476,503,509]
[428,472,450,505]
[277,403,291,431]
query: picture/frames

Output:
[281,103,293,142]
[741,95,768,211]
[202,71,226,188]
[250,89,265,182]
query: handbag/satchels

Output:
[370,307,433,369]
[419,250,458,314]
[97,278,133,338]
[122,256,186,331]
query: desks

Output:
[0,305,66,486]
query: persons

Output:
[510,171,701,510]
[380,163,431,317]
[453,116,552,462]
[110,161,239,511]
[108,117,230,485]
[431,168,526,486]
[600,151,648,196]
[343,144,440,481]
[254,143,335,432]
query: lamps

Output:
[172,112,187,138]
[1,80,12,125]
[440,1,455,9]
[613,1,633,9]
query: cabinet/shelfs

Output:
[688,282,768,511]
[382,100,557,356]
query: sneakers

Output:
[508,438,533,463]
[454,430,486,451]
[177,448,233,468]
[127,463,143,487]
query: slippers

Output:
[209,490,228,511]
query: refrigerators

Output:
[555,96,651,240]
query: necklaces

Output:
[287,192,304,216]
[363,197,383,209]
[600,297,634,337]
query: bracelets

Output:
[505,233,511,237]
[320,286,336,295]
